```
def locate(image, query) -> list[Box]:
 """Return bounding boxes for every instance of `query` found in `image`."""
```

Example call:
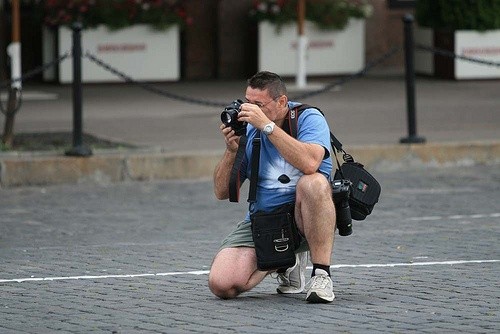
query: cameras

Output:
[331,178,354,235]
[221,98,248,136]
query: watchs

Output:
[262,122,275,136]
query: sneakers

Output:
[276,250,307,293]
[303,268,335,303]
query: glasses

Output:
[246,97,277,108]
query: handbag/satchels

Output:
[250,202,301,271]
[333,161,381,221]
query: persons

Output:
[209,71,337,303]
